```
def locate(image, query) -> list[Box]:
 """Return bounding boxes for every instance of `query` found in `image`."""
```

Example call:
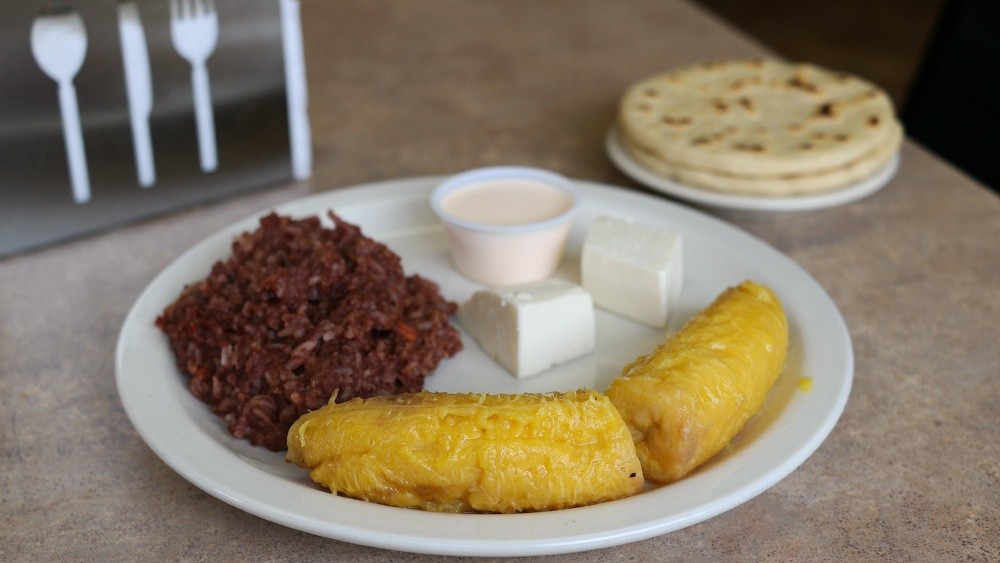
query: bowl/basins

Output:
[429,166,583,283]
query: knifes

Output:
[111,0,159,188]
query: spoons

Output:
[34,14,95,207]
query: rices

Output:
[150,206,478,462]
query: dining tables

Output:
[0,0,1000,563]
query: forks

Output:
[169,1,223,170]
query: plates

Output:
[605,126,900,213]
[114,174,857,555]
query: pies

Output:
[617,57,908,196]
